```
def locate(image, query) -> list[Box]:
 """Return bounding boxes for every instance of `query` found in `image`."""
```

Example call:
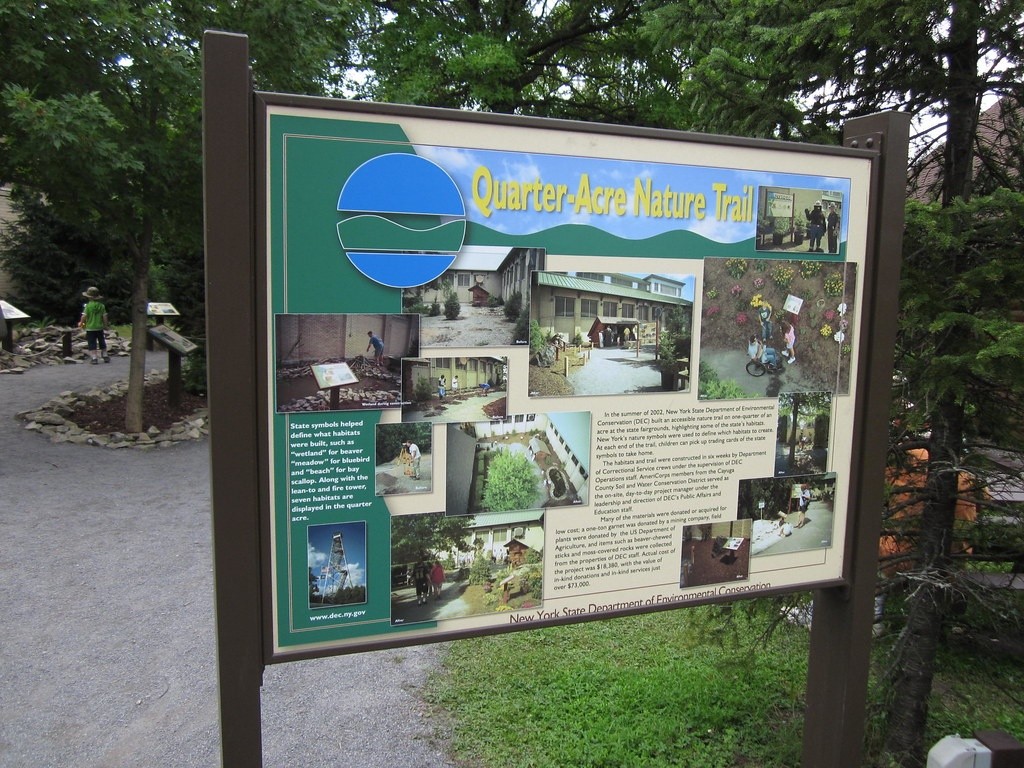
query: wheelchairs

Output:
[746,347,785,377]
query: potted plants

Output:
[659,339,681,392]
[772,210,805,248]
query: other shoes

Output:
[90,358,98,364]
[104,356,110,363]
[788,357,795,364]
[781,350,789,357]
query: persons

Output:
[77,287,110,364]
[605,325,612,345]
[805,200,825,252]
[748,334,777,369]
[781,321,796,364]
[407,439,421,480]
[366,331,384,366]
[795,485,810,528]
[438,375,445,398]
[492,549,503,564]
[780,449,991,638]
[459,553,473,566]
[779,520,791,536]
[452,375,459,394]
[479,383,490,397]
[624,327,630,341]
[827,203,840,253]
[758,300,773,341]
[412,559,446,606]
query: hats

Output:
[83,286,103,300]
